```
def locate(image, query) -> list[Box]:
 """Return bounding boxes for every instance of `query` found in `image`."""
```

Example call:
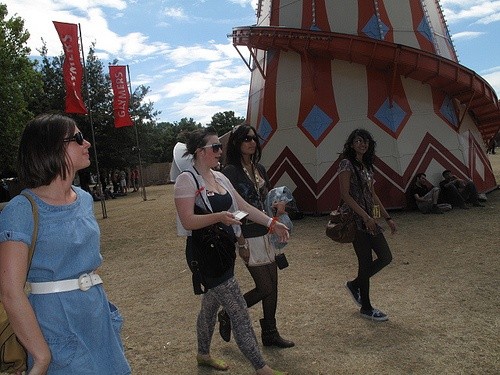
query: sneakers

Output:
[345,281,362,306]
[360,307,389,321]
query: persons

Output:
[218,125,295,347]
[337,128,397,320]
[93,170,139,200]
[411,173,451,213]
[440,170,485,209]
[169,128,290,375]
[0,114,132,375]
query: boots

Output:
[259,318,295,347]
[217,310,230,342]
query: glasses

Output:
[243,134,259,143]
[198,144,224,153]
[354,140,370,145]
[62,132,84,146]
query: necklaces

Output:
[204,177,221,193]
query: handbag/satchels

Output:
[327,210,355,243]
[180,170,236,274]
[0,195,39,375]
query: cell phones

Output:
[232,210,250,221]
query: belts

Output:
[30,272,104,294]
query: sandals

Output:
[272,370,284,375]
[197,356,228,370]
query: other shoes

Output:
[474,201,486,206]
[459,203,469,209]
[422,210,430,214]
[432,204,438,212]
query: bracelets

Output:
[453,181,455,183]
[267,218,273,227]
[364,217,371,223]
[386,217,392,221]
[269,217,276,231]
[239,242,248,249]
